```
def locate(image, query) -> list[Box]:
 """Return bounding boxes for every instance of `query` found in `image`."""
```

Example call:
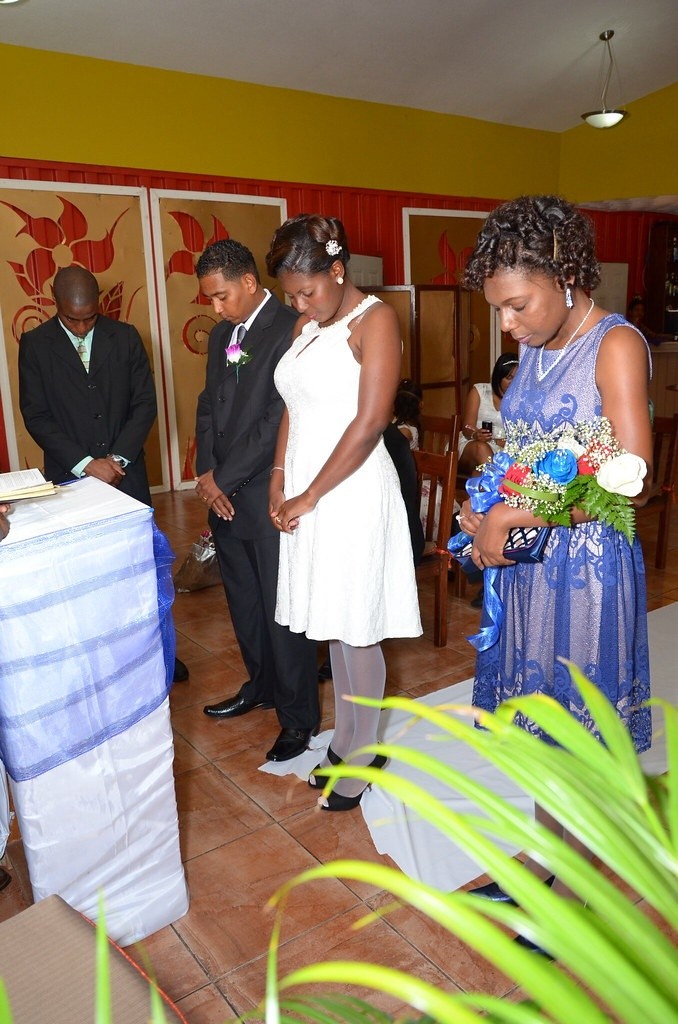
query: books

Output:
[0,467,57,503]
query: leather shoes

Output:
[266,727,318,761]
[469,873,556,904]
[317,659,331,678]
[514,934,556,961]
[203,693,275,717]
[470,587,484,606]
[172,658,189,682]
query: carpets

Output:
[258,602,678,892]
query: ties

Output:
[236,326,247,346]
[77,339,89,374]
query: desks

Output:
[0,476,190,950]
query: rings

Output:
[455,515,467,525]
[276,517,281,523]
[202,496,208,500]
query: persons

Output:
[18,265,188,684]
[192,239,323,763]
[446,194,655,967]
[0,503,11,514]
[457,352,520,492]
[383,378,426,568]
[263,213,424,810]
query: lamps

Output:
[580,30,627,130]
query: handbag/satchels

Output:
[454,525,550,573]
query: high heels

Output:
[307,745,343,789]
[317,751,387,811]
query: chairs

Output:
[411,414,678,649]
[0,894,185,1024]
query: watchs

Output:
[112,454,124,464]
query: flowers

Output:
[222,341,252,384]
[474,413,647,548]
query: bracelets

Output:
[470,432,475,439]
[270,467,285,476]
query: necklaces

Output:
[538,298,594,381]
